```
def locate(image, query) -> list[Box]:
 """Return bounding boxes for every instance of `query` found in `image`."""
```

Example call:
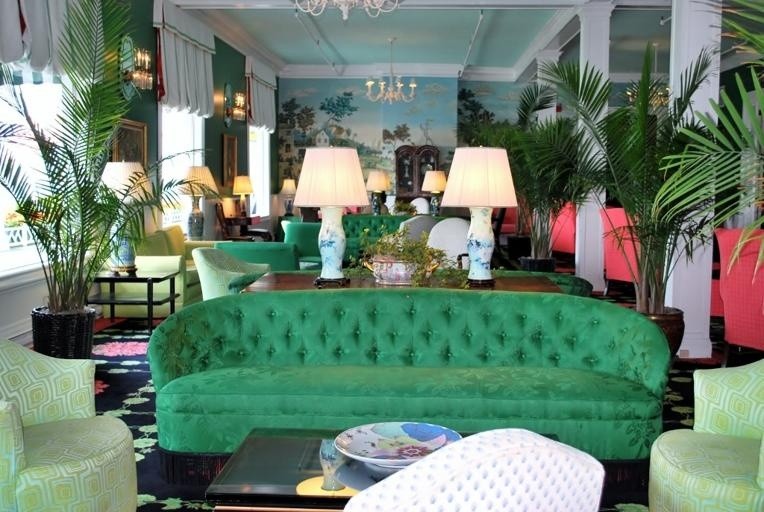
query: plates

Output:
[334,421,462,469]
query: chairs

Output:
[339,426,610,512]
[0,332,139,512]
[474,186,577,257]
[644,356,763,512]
[213,211,474,271]
[214,198,276,243]
[714,224,764,368]
[598,193,653,301]
[191,241,273,304]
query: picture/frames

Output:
[222,132,239,187]
[110,112,149,175]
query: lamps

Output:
[415,166,449,219]
[363,34,419,106]
[89,157,156,276]
[364,166,387,214]
[290,144,373,280]
[626,41,669,112]
[294,0,406,18]
[124,35,155,94]
[436,144,521,286]
[277,176,298,219]
[227,89,249,122]
[178,162,218,241]
[232,173,254,217]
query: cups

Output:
[317,437,345,491]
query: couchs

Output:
[226,272,594,299]
[144,285,672,462]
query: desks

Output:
[239,269,566,297]
[83,268,182,337]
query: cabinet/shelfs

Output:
[394,144,442,212]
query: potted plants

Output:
[516,116,580,275]
[459,106,533,261]
[0,0,202,360]
[345,229,469,286]
[527,38,713,362]
